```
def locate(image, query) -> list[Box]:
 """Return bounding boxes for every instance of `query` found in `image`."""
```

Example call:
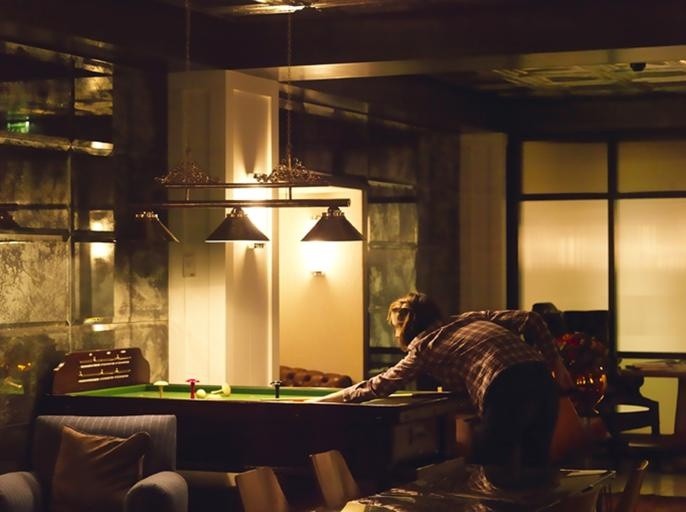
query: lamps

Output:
[126,2,366,251]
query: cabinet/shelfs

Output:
[0,41,124,356]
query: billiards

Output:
[196,389,206,398]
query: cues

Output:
[260,393,455,401]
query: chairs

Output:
[536,306,661,446]
[1,410,189,512]
[227,451,356,511]
[541,471,616,511]
[616,458,649,511]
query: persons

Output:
[308,292,572,466]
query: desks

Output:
[46,376,470,486]
[631,355,685,446]
[318,449,616,511]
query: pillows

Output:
[48,426,150,510]
